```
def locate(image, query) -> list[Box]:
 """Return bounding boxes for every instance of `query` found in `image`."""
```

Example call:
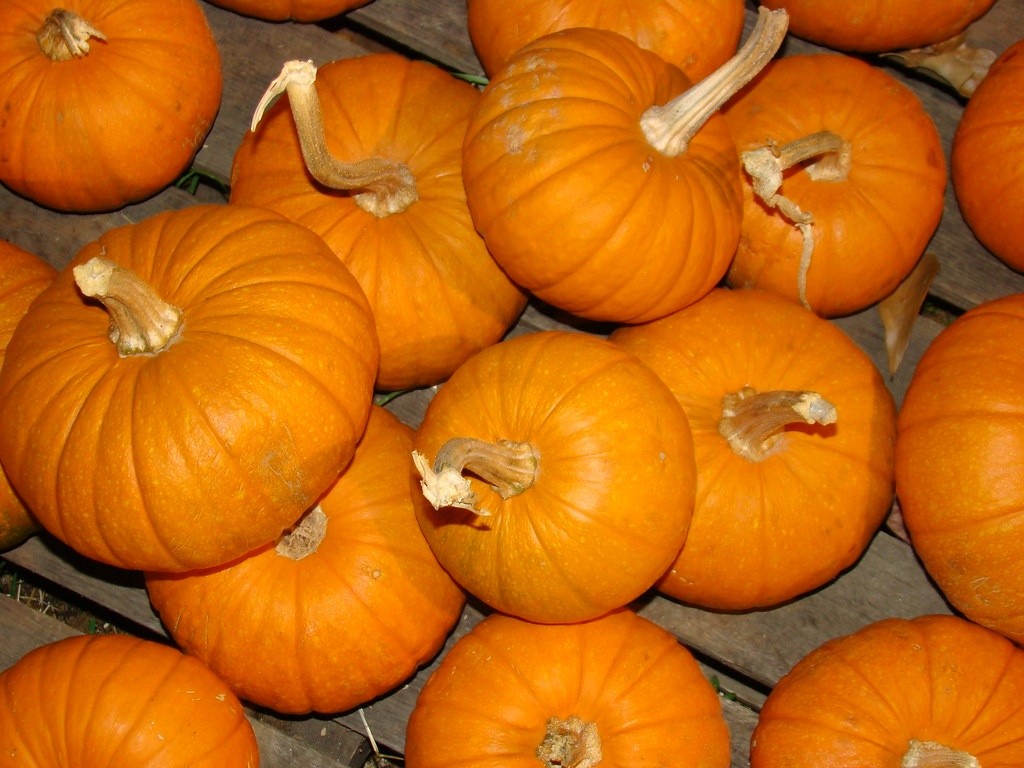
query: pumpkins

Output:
[1,0,1023,768]
